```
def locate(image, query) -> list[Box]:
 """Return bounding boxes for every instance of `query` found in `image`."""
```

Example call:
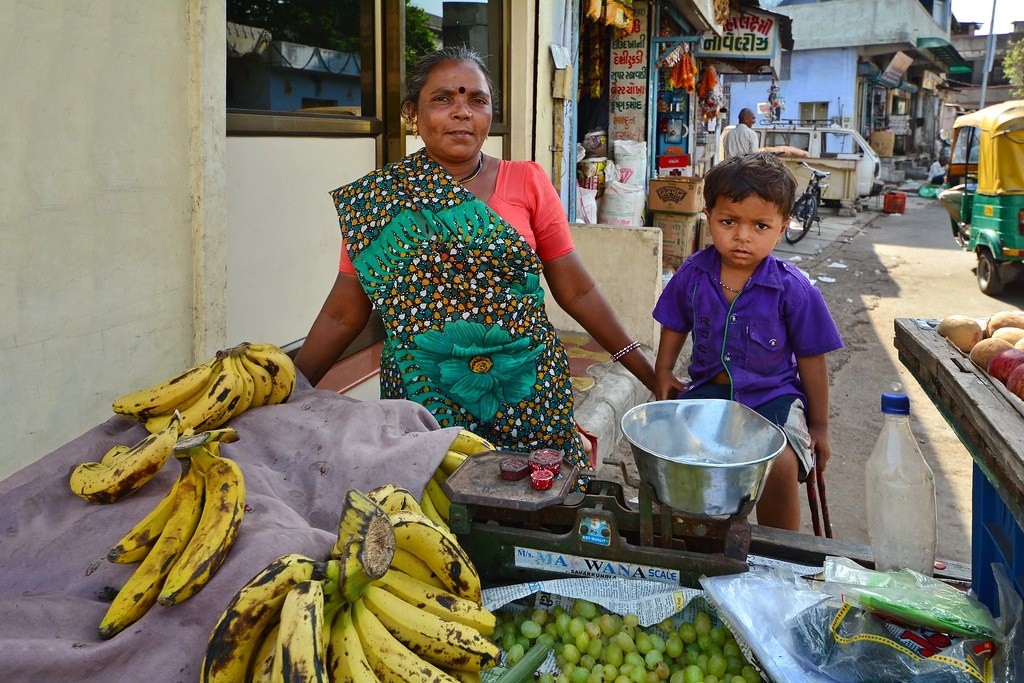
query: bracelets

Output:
[610,340,640,363]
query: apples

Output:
[987,348,1024,402]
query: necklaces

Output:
[452,151,484,184]
[719,281,741,292]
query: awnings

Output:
[917,37,973,72]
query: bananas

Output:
[200,484,503,683]
[70,342,298,641]
[420,428,494,539]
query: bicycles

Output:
[784,161,831,244]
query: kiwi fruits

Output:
[938,311,1024,370]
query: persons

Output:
[291,46,657,493]
[652,154,844,531]
[723,108,758,159]
[928,157,949,184]
[938,183,978,238]
[935,133,952,162]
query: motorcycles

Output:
[939,100,1024,295]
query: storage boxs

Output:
[972,459,1024,618]
[870,131,895,157]
[649,154,715,268]
[884,193,907,213]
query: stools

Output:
[803,450,833,540]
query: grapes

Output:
[502,599,766,683]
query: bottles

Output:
[865,393,939,579]
